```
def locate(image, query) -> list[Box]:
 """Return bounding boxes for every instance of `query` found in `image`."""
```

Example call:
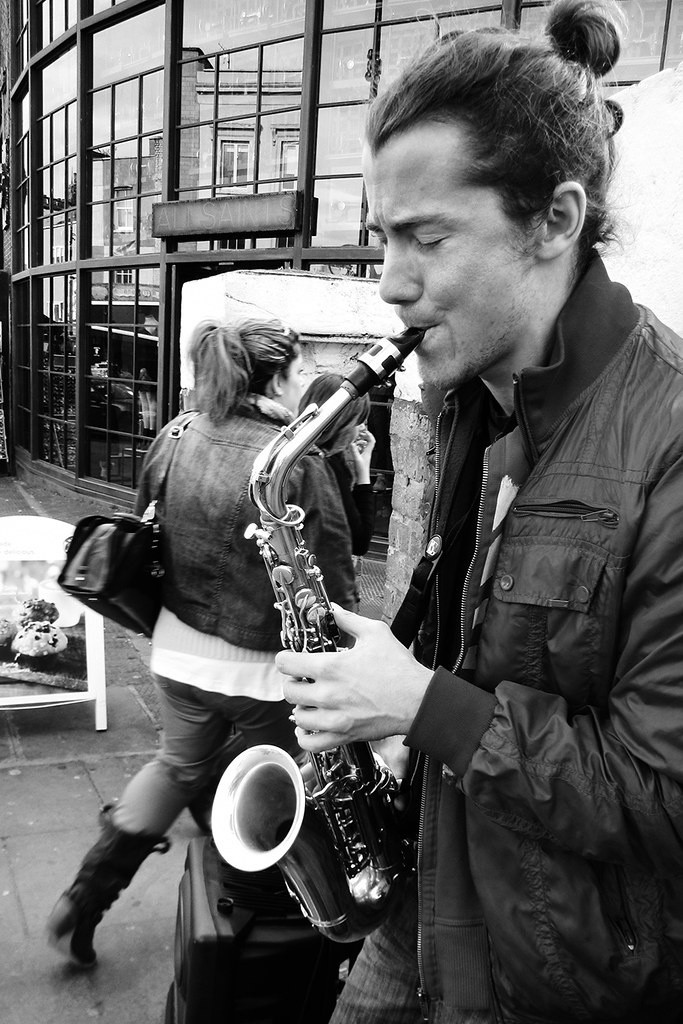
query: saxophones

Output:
[210,328,428,948]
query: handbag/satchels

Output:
[56,410,200,640]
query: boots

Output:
[45,798,171,969]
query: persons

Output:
[272,0,683,1024]
[50,320,359,975]
[296,374,377,646]
